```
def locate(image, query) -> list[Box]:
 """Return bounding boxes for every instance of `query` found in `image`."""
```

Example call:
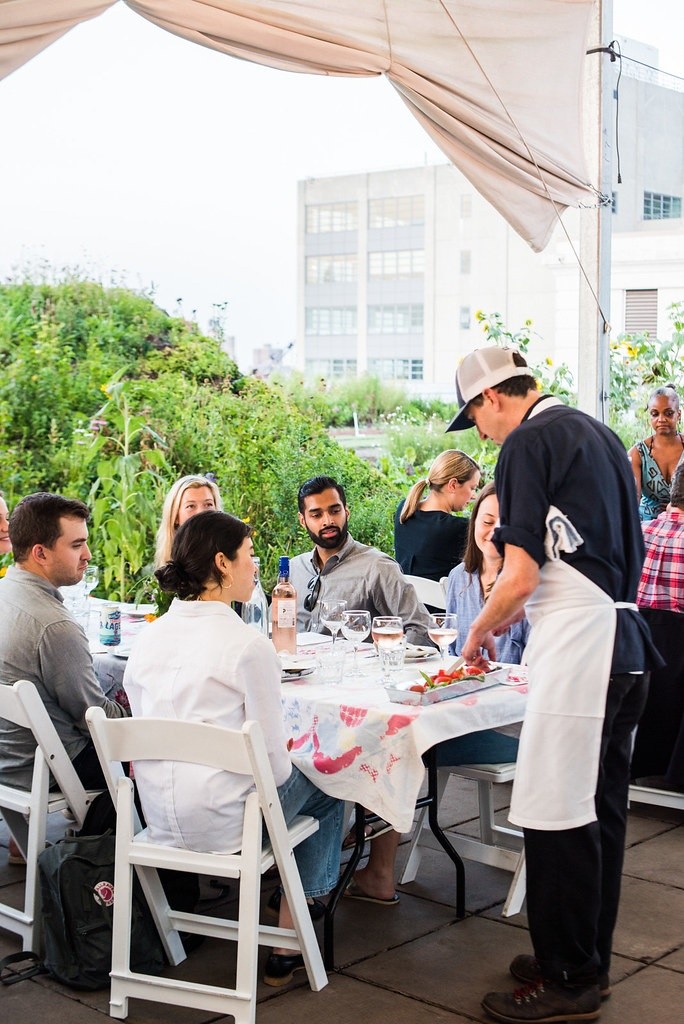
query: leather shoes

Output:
[265,887,326,920]
[510,954,611,997]
[264,945,323,986]
[481,980,602,1024]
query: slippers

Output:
[328,873,400,905]
[341,819,393,852]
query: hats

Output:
[445,346,533,433]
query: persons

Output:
[340,481,529,906]
[156,474,267,623]
[444,344,653,1024]
[635,458,683,614]
[395,449,480,580]
[123,511,346,991]
[266,475,440,653]
[1,494,204,949]
[0,491,13,553]
[630,384,684,519]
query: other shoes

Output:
[65,799,91,820]
[181,935,206,954]
[195,879,230,904]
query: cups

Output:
[317,646,343,683]
[65,580,86,614]
[63,600,91,632]
[378,633,407,671]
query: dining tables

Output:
[68,598,529,971]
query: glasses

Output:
[304,575,321,612]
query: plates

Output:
[106,644,134,658]
[296,631,342,646]
[279,660,317,681]
[405,654,437,663]
[127,609,154,617]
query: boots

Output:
[8,833,54,864]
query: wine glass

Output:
[341,610,372,679]
[82,566,99,609]
[428,612,459,672]
[320,599,348,649]
[371,615,403,690]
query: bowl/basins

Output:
[268,623,272,638]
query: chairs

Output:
[397,724,527,917]
[0,680,107,961]
[85,706,329,1024]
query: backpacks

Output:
[0,829,167,993]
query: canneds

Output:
[98,602,122,647]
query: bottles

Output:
[241,556,269,638]
[272,556,297,658]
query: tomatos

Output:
[408,665,483,692]
